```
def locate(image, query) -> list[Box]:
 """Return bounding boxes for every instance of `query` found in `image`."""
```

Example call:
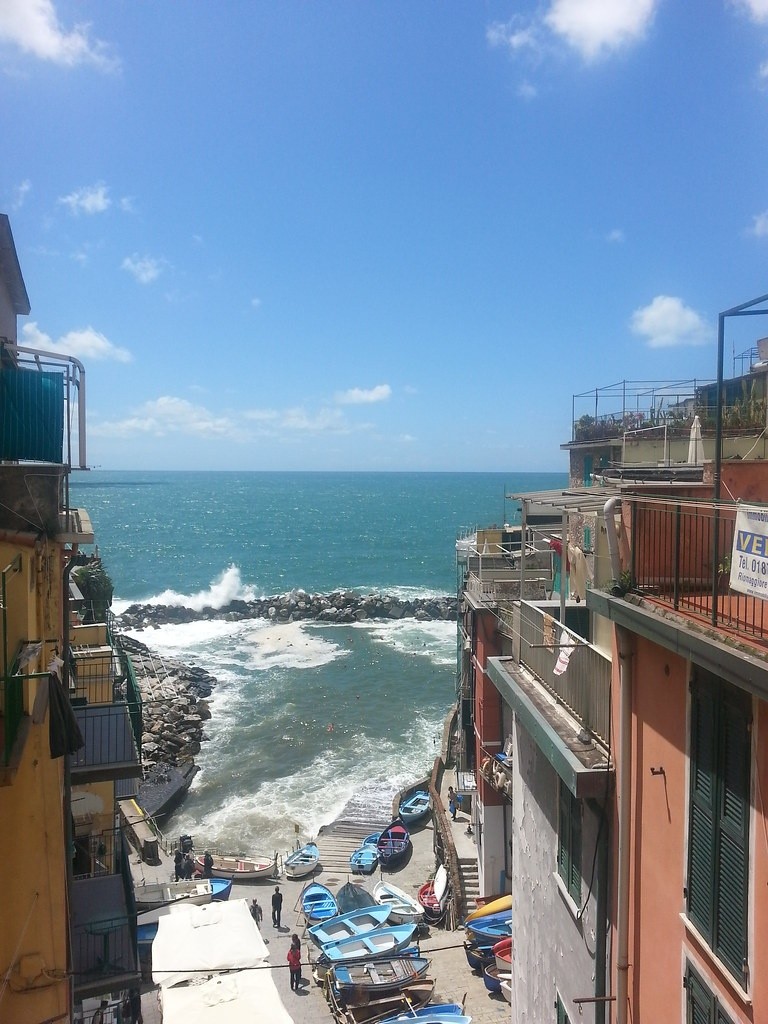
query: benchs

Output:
[391,904,410,909]
[421,894,436,898]
[302,899,333,905]
[366,963,380,984]
[298,858,314,860]
[381,897,399,902]
[380,838,407,842]
[357,858,377,861]
[423,901,439,903]
[292,861,308,864]
[404,805,425,810]
[341,919,362,935]
[163,888,171,900]
[390,961,404,978]
[196,884,205,896]
[361,937,378,953]
[305,907,335,913]
[418,796,429,800]
[359,851,376,854]
[378,845,403,849]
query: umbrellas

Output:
[687,417,704,462]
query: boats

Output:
[133,877,234,912]
[418,874,454,917]
[336,875,378,914]
[313,944,473,1024]
[362,831,384,849]
[373,871,425,925]
[349,843,380,874]
[306,904,392,945]
[193,853,277,880]
[299,876,339,927]
[398,789,431,825]
[321,922,418,966]
[463,891,512,1005]
[376,816,411,869]
[283,842,321,878]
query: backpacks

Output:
[251,905,259,920]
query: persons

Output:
[448,786,458,821]
[272,886,282,927]
[184,854,195,881]
[174,849,184,881]
[249,898,262,924]
[287,943,300,990]
[292,934,300,949]
[202,849,213,878]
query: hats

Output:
[203,849,209,855]
